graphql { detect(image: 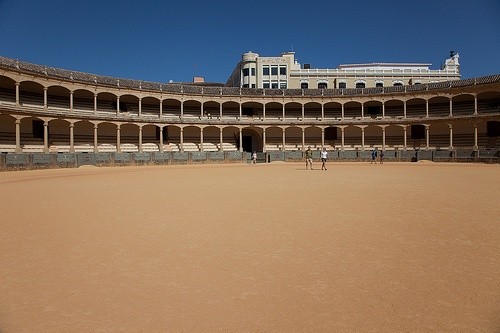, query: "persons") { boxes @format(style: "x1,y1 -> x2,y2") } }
369,148 -> 377,164
250,152 -> 254,165
253,150 -> 257,165
380,150 -> 384,164
320,147 -> 328,170
305,146 -> 315,170
